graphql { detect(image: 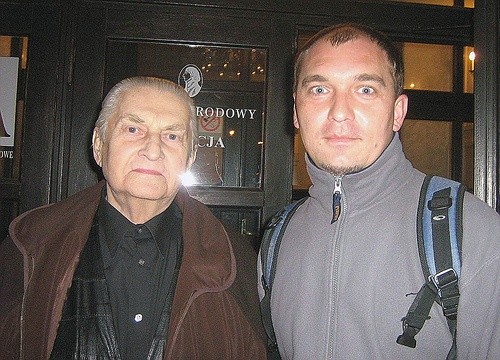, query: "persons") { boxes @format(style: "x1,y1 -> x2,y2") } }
256,22 -> 500,359
0,76 -> 271,359
